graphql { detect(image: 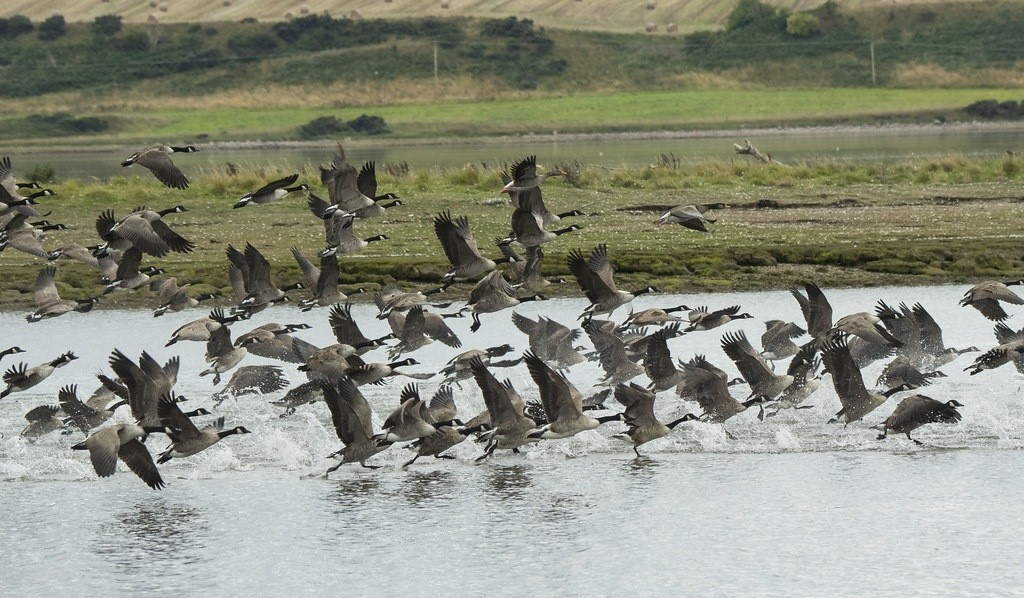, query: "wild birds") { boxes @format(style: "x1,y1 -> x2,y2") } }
0,144 -> 1024,491
